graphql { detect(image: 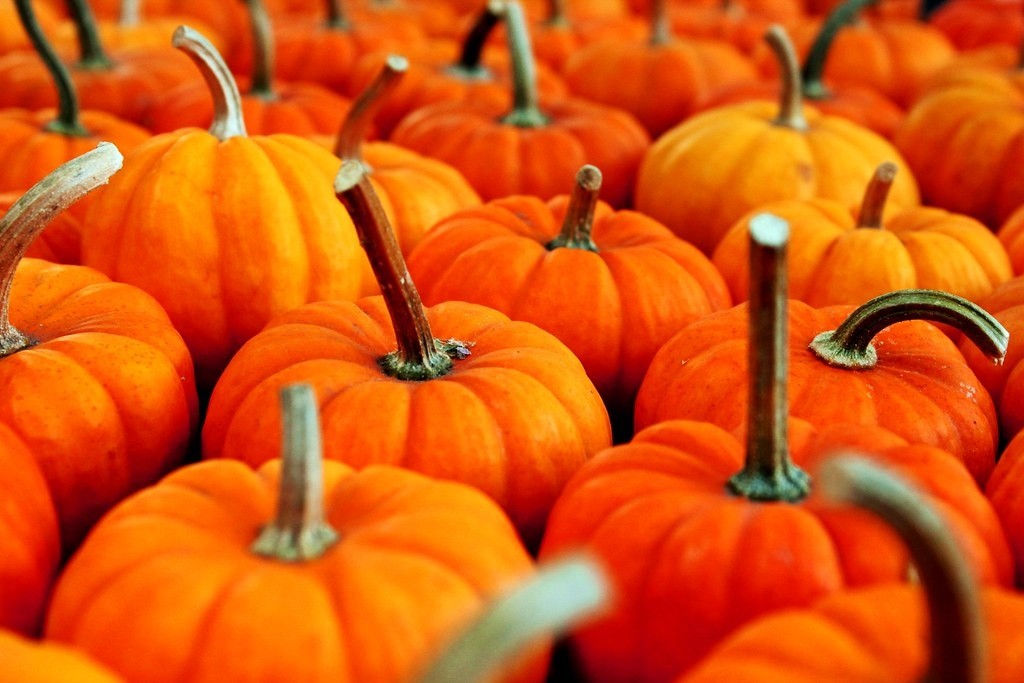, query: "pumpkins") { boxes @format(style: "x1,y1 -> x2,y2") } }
0,0 -> 1024,683
